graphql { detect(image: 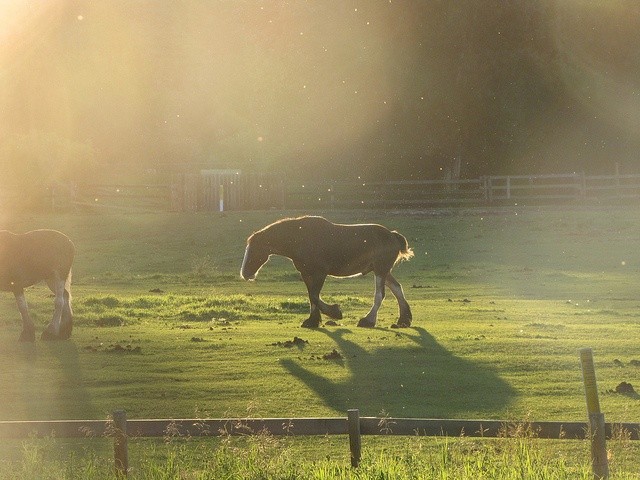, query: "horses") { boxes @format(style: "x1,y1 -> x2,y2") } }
1,229 -> 75,343
240,214 -> 415,331
